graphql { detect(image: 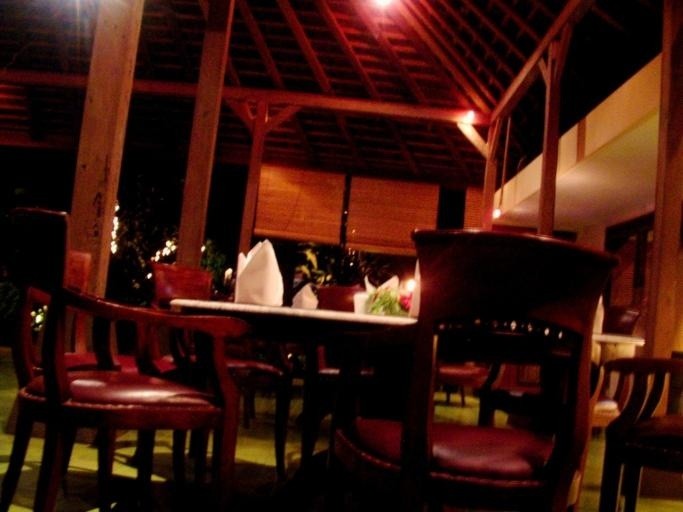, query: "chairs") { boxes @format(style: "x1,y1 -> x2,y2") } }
0,207 -> 683,512
